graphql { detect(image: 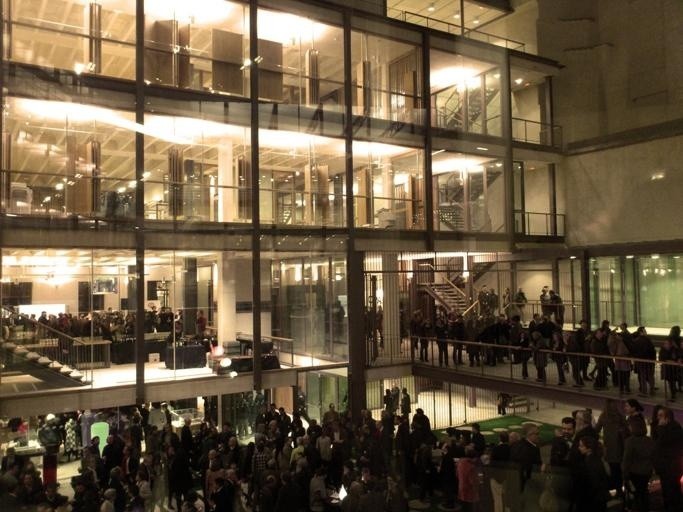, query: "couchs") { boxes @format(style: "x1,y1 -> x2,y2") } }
6,324 -> 284,378
